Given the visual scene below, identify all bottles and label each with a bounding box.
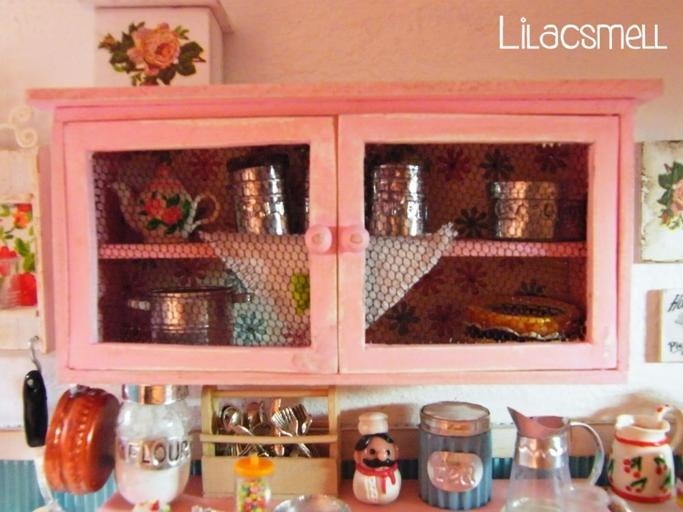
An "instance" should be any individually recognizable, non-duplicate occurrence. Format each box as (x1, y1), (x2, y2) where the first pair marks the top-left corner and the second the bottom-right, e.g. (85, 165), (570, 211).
(415, 396), (494, 510)
(505, 405), (606, 512)
(233, 451), (274, 512)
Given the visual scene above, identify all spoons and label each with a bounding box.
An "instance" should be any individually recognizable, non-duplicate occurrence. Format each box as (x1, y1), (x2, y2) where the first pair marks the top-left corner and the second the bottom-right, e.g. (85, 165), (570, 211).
(220, 400), (277, 456)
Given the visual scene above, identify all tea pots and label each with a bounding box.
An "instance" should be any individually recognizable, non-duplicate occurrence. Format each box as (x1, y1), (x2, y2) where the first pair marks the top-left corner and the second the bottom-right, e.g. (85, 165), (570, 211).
(605, 403), (683, 504)
(105, 161), (221, 243)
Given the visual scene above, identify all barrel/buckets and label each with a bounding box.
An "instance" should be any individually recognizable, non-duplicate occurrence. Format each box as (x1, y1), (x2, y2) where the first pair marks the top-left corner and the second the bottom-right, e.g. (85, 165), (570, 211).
(226, 153), (291, 235)
(366, 161), (429, 236)
(488, 180), (560, 242)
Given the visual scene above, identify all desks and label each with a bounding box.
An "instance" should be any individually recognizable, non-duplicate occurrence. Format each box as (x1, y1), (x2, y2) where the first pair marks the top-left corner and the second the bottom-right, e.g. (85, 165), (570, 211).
(96, 476), (682, 511)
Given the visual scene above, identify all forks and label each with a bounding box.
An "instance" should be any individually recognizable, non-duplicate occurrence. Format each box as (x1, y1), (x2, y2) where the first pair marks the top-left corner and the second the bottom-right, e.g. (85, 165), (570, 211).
(270, 403), (314, 458)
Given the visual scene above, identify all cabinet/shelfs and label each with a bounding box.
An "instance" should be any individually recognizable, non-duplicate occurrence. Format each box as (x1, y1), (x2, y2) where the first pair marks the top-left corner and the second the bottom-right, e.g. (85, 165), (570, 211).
(25, 78), (665, 389)
